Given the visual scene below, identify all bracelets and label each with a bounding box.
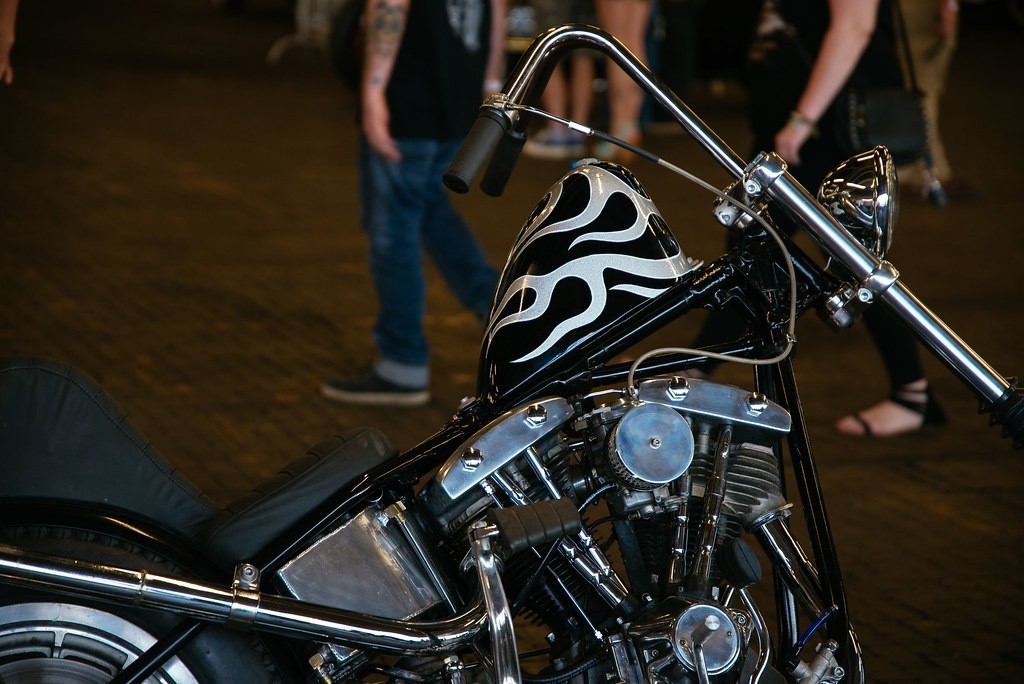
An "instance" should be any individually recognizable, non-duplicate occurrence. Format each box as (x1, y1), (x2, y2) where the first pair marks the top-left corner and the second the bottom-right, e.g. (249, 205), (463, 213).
(784, 108), (822, 138)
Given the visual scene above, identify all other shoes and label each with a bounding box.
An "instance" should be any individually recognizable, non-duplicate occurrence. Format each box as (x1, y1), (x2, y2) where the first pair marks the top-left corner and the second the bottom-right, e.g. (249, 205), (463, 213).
(591, 125), (641, 161)
(520, 126), (587, 160)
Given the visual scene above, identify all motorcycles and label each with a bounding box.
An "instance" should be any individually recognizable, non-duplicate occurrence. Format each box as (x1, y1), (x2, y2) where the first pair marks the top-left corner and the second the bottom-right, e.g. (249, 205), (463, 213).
(0, 22), (1024, 684)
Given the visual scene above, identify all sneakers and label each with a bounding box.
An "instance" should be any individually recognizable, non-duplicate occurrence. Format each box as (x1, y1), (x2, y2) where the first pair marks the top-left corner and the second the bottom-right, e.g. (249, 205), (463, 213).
(320, 366), (432, 407)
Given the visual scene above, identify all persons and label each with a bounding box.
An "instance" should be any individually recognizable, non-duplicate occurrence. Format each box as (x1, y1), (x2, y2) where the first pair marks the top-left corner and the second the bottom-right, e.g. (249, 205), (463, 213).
(263, 0), (972, 438)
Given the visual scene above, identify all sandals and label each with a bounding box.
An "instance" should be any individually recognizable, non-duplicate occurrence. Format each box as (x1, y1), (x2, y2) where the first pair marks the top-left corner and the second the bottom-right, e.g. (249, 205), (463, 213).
(833, 388), (948, 440)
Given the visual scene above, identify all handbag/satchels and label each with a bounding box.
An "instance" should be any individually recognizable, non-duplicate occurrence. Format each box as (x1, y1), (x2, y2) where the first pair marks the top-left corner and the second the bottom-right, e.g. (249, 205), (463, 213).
(833, 91), (928, 166)
